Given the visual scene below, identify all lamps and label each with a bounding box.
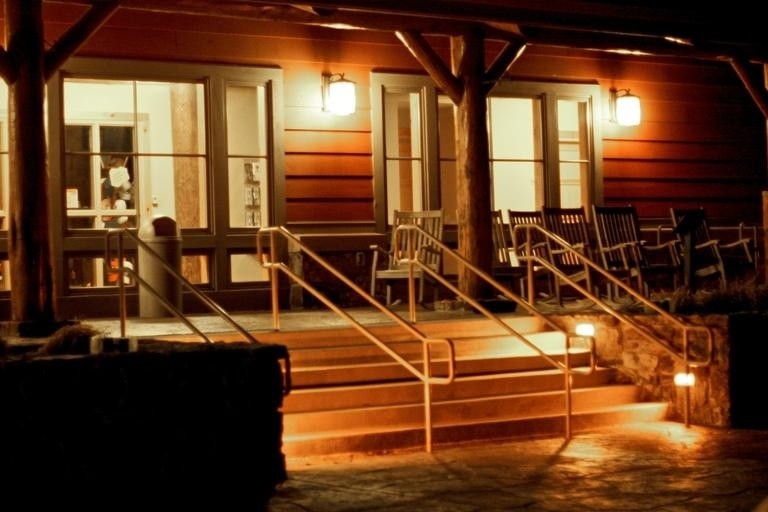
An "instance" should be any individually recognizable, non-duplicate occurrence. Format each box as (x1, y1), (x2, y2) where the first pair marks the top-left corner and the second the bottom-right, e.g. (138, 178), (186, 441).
(321, 71), (358, 116)
(608, 86), (642, 127)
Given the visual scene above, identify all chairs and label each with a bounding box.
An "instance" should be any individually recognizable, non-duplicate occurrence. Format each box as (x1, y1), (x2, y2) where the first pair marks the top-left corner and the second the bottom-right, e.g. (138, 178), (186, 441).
(369, 208), (445, 305)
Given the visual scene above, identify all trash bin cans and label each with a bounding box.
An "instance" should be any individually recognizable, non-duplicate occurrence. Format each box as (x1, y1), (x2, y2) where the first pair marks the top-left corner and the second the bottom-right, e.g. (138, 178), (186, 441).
(137, 215), (183, 320)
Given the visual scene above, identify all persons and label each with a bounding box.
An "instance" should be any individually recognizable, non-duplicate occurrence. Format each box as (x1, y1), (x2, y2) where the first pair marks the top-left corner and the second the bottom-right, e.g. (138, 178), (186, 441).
(33, 322), (120, 355)
